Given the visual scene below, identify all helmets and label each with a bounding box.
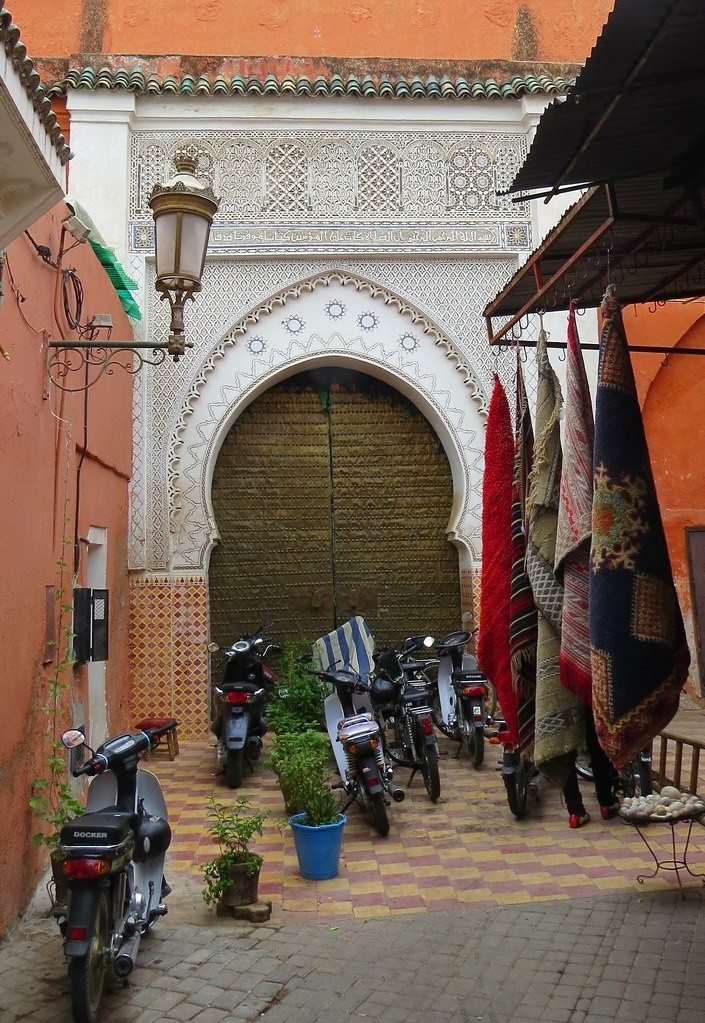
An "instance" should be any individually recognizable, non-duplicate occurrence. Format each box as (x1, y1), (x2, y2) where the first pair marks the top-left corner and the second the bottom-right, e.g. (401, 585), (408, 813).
(132, 814), (171, 863)
(370, 675), (397, 704)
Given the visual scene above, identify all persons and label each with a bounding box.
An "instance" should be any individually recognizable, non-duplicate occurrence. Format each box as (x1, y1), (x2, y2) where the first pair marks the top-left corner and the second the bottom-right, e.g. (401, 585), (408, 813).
(553, 728), (619, 827)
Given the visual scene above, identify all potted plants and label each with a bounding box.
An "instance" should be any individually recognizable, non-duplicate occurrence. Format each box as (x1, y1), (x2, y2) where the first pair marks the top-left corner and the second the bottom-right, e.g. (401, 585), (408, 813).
(198, 787), (271, 907)
(27, 491), (88, 905)
(262, 636), (330, 817)
(261, 728), (349, 881)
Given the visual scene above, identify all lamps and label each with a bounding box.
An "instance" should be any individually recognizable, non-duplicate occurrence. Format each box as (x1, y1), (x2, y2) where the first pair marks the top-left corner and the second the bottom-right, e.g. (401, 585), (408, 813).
(41, 147), (222, 401)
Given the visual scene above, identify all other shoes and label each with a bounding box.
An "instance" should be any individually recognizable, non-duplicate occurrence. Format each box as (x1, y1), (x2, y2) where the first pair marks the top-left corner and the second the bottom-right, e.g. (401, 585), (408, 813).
(600, 803), (620, 820)
(569, 812), (590, 828)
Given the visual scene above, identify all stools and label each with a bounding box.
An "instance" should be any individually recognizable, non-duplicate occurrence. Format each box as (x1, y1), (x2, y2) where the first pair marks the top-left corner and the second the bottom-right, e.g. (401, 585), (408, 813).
(135, 718), (179, 762)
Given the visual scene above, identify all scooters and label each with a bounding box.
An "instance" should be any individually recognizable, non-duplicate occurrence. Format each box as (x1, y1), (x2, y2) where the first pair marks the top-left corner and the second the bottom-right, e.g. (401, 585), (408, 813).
(368, 611), (490, 803)
(207, 634), (280, 790)
(489, 708), (654, 825)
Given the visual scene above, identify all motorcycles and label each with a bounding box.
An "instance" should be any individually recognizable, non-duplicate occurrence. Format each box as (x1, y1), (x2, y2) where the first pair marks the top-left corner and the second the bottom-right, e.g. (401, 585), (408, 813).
(307, 659), (405, 838)
(46, 717), (180, 1023)
